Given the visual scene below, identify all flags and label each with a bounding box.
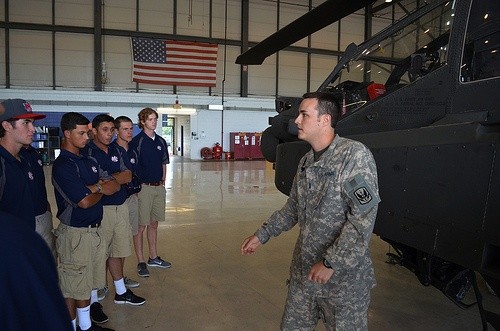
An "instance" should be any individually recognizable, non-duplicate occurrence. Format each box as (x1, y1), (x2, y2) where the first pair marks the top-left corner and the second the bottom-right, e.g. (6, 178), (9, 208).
(131, 35), (217, 87)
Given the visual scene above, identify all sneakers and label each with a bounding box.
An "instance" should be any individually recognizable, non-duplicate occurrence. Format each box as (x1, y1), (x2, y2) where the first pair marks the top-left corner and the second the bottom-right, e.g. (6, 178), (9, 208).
(147, 256), (172, 268)
(137, 262), (150, 276)
(114, 288), (145, 306)
(124, 277), (140, 287)
(97, 287), (109, 300)
(90, 302), (109, 324)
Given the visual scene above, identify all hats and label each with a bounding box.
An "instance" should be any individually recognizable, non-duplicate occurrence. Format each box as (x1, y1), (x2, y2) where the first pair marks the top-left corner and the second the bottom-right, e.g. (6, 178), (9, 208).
(0, 98), (46, 122)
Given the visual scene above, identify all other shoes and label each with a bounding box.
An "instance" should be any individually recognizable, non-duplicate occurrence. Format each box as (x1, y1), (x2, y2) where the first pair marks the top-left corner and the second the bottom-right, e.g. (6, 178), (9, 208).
(76, 321), (115, 331)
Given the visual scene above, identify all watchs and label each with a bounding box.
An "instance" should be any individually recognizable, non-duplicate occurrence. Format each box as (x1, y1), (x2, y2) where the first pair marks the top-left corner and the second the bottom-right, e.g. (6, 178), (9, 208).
(322, 258), (333, 269)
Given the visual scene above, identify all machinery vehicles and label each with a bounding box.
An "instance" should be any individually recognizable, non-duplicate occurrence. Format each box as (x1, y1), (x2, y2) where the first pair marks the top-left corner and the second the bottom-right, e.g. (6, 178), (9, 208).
(235, 0), (500, 331)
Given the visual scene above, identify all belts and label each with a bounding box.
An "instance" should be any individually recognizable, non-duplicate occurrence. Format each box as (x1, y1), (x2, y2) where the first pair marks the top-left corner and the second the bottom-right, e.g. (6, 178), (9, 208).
(144, 181), (163, 186)
(89, 220), (102, 230)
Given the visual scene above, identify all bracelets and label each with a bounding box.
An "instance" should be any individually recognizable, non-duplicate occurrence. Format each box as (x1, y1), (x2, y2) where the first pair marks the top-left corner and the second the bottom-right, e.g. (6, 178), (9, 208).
(96, 184), (102, 193)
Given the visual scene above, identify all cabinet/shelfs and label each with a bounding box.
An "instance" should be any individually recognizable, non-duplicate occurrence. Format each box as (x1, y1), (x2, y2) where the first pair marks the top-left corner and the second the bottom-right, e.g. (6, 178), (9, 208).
(230, 131), (265, 162)
(33, 126), (60, 166)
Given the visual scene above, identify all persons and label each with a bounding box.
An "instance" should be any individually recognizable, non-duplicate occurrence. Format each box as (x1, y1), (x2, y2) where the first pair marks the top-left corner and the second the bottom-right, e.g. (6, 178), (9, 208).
(97, 115), (139, 301)
(0, 211), (74, 331)
(50, 112), (120, 331)
(129, 107), (172, 277)
(0, 98), (47, 232)
(89, 114), (146, 324)
(240, 92), (382, 331)
(18, 143), (58, 263)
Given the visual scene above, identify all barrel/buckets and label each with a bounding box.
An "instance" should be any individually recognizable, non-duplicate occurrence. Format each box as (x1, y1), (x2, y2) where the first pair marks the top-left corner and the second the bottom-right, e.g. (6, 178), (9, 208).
(226, 153), (231, 160)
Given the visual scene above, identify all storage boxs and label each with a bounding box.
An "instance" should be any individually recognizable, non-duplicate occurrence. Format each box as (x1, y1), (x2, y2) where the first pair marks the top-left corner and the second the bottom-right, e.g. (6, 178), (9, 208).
(366, 83), (386, 101)
